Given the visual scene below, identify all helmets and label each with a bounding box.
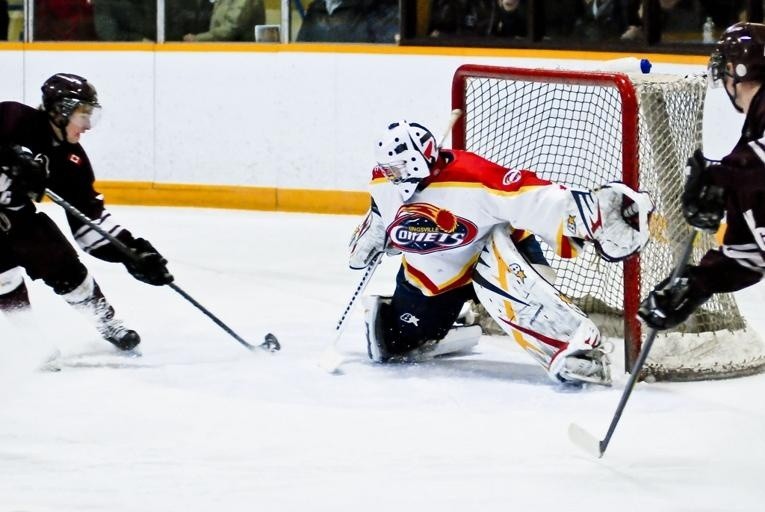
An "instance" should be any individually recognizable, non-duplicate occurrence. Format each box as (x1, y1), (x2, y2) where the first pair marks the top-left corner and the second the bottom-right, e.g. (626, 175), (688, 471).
(706, 20), (764, 112)
(42, 70), (102, 133)
(375, 119), (437, 202)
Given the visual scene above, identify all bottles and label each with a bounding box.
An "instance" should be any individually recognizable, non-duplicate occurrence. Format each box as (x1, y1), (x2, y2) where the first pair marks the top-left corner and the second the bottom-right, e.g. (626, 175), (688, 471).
(702, 17), (716, 44)
(593, 56), (654, 76)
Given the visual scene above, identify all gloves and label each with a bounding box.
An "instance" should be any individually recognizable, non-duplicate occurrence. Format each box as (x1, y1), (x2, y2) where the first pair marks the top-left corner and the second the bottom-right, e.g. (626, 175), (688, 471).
(681, 147), (729, 235)
(635, 262), (715, 331)
(11, 146), (46, 202)
(124, 235), (174, 284)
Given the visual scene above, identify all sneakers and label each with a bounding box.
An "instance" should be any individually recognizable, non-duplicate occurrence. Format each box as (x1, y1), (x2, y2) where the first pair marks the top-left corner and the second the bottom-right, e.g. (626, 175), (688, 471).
(99, 319), (140, 352)
(557, 352), (602, 388)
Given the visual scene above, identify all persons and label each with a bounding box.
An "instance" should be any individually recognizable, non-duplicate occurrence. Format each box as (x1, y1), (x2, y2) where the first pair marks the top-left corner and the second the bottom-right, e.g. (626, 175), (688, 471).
(295, 2), (398, 42)
(632, 21), (765, 331)
(427, 3), (762, 48)
(0, 4), (266, 41)
(0, 72), (175, 352)
(337, 117), (659, 392)
